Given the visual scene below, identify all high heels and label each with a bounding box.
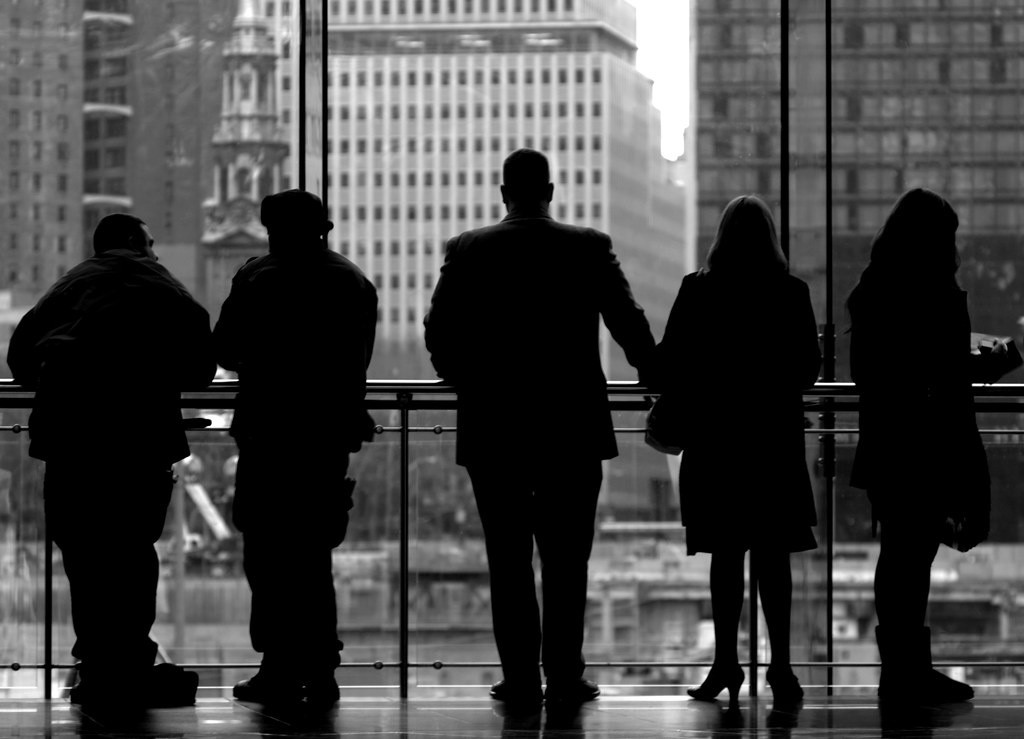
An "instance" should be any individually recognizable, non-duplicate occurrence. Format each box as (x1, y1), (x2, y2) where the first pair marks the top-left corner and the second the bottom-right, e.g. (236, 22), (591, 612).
(765, 667), (803, 705)
(689, 662), (744, 704)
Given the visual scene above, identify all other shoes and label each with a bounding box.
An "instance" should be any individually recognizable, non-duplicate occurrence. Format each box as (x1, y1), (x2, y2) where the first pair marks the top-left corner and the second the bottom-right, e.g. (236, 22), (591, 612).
(545, 676), (597, 700)
(493, 679), (544, 703)
(923, 668), (974, 705)
(234, 681), (299, 707)
(877, 688), (954, 727)
(304, 673), (339, 702)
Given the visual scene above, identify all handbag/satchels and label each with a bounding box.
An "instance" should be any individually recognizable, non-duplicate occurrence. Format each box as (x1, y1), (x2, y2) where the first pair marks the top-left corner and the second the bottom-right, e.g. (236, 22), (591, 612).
(645, 383), (697, 455)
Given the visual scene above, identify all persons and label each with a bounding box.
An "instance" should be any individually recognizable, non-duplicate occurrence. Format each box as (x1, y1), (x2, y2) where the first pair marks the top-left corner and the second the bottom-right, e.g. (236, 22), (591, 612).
(7, 213), (217, 702)
(659, 197), (822, 704)
(423, 150), (656, 703)
(213, 189), (378, 700)
(845, 189), (1008, 704)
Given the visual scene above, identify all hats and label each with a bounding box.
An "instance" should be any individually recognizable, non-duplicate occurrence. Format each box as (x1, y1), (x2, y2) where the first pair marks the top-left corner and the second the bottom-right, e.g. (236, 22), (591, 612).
(259, 189), (333, 234)
